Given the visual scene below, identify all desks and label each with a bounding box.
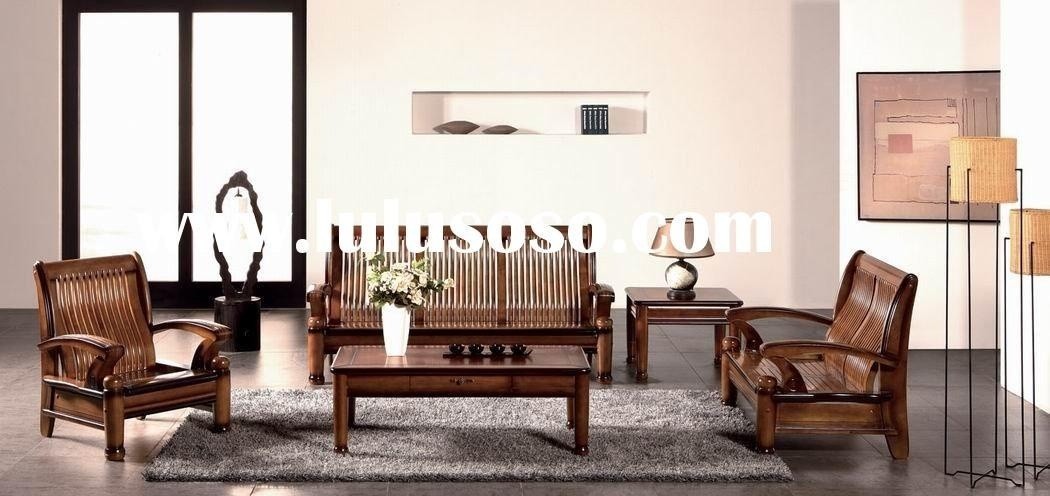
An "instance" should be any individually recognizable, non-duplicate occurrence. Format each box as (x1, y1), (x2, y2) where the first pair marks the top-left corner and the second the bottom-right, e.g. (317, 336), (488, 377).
(623, 284), (746, 383)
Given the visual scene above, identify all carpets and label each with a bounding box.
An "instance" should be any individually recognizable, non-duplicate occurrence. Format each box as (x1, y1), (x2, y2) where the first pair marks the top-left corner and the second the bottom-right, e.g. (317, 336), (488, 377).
(142, 382), (798, 486)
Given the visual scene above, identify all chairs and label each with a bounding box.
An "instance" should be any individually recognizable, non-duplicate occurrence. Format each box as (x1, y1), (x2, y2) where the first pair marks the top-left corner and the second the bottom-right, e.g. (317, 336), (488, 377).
(30, 249), (236, 463)
(717, 248), (920, 463)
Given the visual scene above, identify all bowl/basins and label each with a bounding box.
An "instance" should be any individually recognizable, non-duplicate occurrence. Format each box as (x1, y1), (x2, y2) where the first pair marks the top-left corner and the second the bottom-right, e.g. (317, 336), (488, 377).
(468, 344), (484, 353)
(510, 343), (527, 353)
(449, 344), (465, 353)
(489, 343), (506, 353)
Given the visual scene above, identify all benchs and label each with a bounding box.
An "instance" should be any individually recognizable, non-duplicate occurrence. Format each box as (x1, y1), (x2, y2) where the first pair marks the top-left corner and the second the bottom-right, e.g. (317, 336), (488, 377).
(303, 213), (617, 391)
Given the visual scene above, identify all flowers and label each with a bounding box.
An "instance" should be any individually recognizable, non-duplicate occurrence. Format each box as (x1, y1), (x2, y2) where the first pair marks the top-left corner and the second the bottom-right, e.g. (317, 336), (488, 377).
(358, 248), (458, 309)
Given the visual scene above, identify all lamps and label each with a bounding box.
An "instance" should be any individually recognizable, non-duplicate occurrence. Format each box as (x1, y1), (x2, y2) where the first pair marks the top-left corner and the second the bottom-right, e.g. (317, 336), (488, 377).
(1000, 202), (1050, 478)
(937, 129), (1025, 489)
(646, 212), (718, 292)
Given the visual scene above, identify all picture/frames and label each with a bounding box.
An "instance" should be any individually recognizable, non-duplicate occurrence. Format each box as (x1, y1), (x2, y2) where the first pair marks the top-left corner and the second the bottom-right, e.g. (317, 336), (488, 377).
(853, 66), (1000, 228)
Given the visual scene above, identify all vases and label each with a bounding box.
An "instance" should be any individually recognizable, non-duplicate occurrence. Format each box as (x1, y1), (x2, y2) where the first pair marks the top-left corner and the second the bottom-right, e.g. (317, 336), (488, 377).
(377, 294), (413, 357)
